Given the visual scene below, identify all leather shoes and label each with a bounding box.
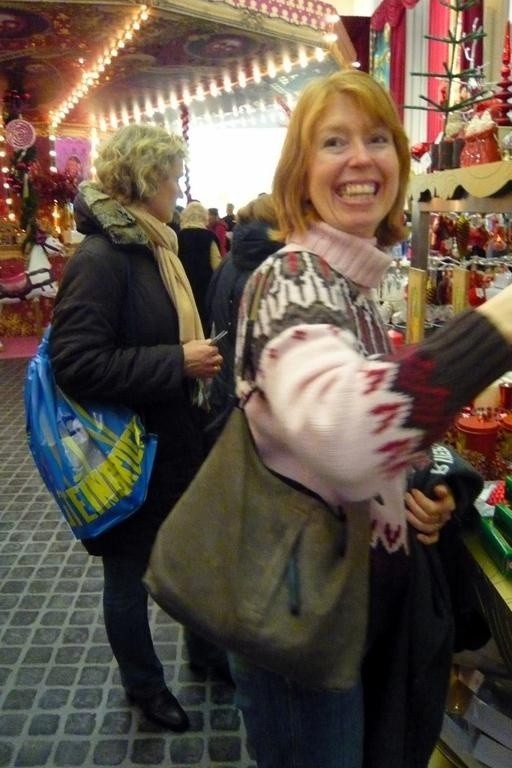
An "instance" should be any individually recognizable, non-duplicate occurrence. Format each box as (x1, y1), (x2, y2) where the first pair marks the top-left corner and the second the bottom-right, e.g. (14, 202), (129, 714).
(125, 685), (189, 733)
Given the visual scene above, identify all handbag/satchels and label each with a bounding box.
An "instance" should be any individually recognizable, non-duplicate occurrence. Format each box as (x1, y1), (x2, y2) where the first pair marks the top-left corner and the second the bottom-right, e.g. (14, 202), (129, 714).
(143, 400), (371, 696)
(22, 322), (161, 541)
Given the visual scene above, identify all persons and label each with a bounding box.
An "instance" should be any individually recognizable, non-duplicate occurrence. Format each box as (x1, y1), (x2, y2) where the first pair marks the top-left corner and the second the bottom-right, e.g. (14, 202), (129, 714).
(48, 123), (238, 733)
(230, 72), (512, 768)
(171, 192), (284, 404)
